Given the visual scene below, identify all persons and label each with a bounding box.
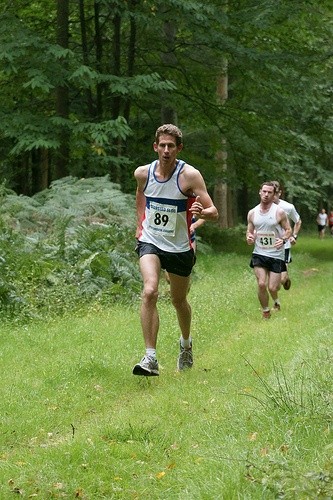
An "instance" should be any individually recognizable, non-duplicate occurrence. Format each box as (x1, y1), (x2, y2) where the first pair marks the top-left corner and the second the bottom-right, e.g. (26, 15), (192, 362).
(133, 124), (219, 375)
(247, 180), (302, 319)
(317, 208), (328, 238)
(328, 211), (333, 234)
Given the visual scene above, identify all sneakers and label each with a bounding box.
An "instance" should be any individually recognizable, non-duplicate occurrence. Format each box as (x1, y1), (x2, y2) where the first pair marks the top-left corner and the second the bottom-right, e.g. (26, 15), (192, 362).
(270, 302), (280, 311)
(262, 310), (270, 319)
(178, 337), (193, 370)
(283, 278), (291, 290)
(132, 355), (159, 376)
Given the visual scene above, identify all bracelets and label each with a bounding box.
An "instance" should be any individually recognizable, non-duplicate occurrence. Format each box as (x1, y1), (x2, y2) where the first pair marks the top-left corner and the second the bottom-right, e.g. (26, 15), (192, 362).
(291, 235), (298, 240)
(281, 237), (286, 245)
